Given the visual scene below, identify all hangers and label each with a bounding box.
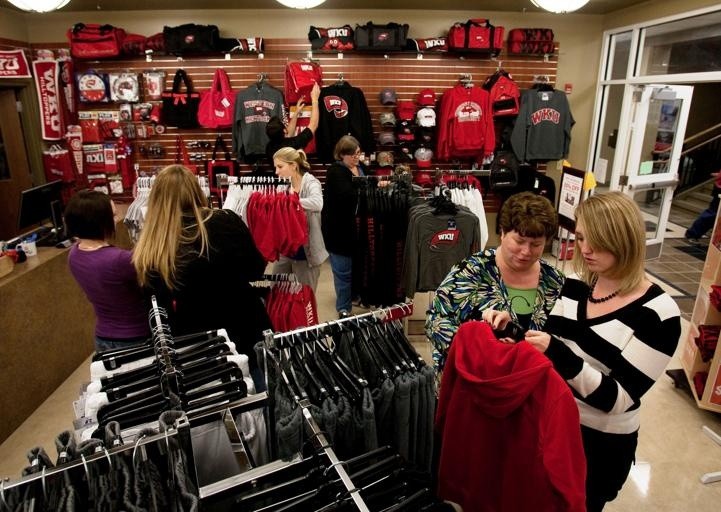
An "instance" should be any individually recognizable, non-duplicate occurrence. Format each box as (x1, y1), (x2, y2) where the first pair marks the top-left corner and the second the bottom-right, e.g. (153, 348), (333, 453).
(136, 174), (292, 209)
(460, 73), (475, 90)
(529, 74), (556, 95)
(0, 295), (443, 512)
(352, 166), (492, 217)
(490, 294), (531, 342)
(255, 73), (265, 87)
(253, 267), (305, 295)
(334, 72), (346, 87)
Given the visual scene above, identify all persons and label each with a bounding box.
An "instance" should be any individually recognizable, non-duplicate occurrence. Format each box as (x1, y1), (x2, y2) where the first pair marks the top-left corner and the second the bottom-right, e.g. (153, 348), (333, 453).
(65, 190), (152, 347)
(321, 137), (372, 318)
(422, 195), (562, 388)
(268, 149), (327, 297)
(133, 164), (277, 387)
(526, 190), (681, 512)
(682, 168), (721, 245)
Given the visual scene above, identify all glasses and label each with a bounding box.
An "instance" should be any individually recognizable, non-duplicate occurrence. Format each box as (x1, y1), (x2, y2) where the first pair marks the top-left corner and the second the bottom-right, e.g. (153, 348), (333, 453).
(343, 151), (360, 157)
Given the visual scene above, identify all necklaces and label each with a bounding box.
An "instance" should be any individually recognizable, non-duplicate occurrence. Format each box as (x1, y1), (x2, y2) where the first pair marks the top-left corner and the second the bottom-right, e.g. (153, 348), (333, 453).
(589, 273), (623, 304)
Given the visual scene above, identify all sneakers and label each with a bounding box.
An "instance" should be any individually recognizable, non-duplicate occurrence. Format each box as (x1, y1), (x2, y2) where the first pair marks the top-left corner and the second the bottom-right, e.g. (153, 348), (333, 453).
(685, 236), (700, 245)
(700, 234), (709, 238)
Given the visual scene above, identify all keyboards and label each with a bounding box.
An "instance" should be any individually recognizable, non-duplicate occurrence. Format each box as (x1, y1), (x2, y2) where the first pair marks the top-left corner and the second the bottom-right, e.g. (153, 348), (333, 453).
(6, 227), (55, 250)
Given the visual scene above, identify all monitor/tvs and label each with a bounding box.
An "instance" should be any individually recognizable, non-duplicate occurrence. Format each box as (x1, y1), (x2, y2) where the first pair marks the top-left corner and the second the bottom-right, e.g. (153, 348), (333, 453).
(18, 179), (73, 248)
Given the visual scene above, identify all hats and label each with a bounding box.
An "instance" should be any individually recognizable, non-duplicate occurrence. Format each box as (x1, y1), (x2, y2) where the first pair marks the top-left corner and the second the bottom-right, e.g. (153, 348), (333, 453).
(379, 133), (395, 145)
(377, 153), (394, 167)
(394, 122), (415, 142)
(417, 90), (437, 105)
(417, 134), (432, 147)
(374, 170), (395, 187)
(418, 107), (436, 127)
(416, 172), (432, 184)
(381, 89), (397, 105)
(394, 100), (417, 121)
(414, 149), (433, 168)
(379, 113), (396, 128)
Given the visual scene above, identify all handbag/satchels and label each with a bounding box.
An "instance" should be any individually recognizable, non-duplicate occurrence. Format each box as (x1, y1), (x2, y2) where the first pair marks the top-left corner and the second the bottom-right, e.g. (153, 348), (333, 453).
(125, 32), (165, 55)
(163, 24), (220, 55)
(308, 25), (354, 50)
(67, 22), (125, 60)
(197, 69), (239, 128)
(227, 38), (264, 53)
(508, 28), (555, 56)
(354, 22), (409, 50)
(449, 18), (504, 50)
(208, 138), (238, 196)
(162, 70), (200, 129)
(409, 37), (450, 50)
